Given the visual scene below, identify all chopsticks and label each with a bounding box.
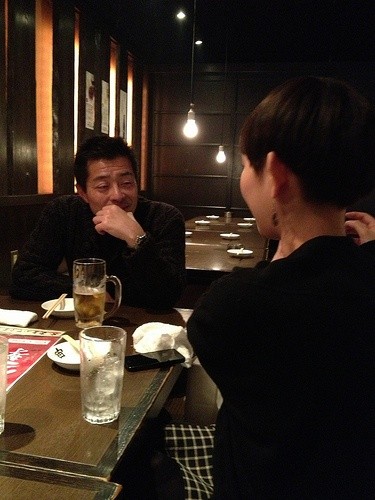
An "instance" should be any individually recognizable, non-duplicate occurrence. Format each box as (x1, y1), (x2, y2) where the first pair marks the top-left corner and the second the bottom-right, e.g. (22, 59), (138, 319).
(237, 247), (244, 255)
(60, 335), (80, 352)
(42, 294), (67, 319)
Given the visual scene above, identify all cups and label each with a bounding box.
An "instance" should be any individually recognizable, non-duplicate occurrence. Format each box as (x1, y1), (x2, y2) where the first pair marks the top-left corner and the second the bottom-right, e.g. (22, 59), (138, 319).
(78, 325), (127, 425)
(0, 334), (9, 434)
(73, 257), (122, 330)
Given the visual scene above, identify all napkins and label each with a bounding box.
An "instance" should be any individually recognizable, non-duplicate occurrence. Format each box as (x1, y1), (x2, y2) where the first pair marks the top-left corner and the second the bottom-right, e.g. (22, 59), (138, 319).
(0, 309), (38, 328)
(131, 322), (194, 368)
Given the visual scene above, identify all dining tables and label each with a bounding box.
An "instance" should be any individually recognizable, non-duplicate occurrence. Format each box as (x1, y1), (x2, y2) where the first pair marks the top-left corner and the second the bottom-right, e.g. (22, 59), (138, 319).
(0, 291), (188, 482)
(184, 215), (270, 307)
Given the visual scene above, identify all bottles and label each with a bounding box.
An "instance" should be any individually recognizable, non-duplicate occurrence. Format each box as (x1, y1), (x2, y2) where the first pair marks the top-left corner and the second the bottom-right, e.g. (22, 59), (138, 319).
(224, 207), (231, 225)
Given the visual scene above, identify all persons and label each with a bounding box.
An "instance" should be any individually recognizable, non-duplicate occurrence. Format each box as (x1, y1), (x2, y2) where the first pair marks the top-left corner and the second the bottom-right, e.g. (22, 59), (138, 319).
(146, 77), (375, 500)
(9, 137), (186, 312)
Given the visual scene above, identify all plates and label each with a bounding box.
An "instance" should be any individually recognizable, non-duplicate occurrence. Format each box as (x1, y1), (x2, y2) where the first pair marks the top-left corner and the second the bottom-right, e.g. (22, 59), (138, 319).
(206, 216), (220, 220)
(237, 223), (254, 229)
(220, 233), (240, 239)
(185, 231), (192, 237)
(195, 220), (211, 226)
(226, 248), (254, 257)
(244, 218), (256, 221)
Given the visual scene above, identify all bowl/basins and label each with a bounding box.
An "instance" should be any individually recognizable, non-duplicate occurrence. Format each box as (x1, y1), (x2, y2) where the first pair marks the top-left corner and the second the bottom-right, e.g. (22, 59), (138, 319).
(42, 298), (75, 318)
(47, 337), (111, 371)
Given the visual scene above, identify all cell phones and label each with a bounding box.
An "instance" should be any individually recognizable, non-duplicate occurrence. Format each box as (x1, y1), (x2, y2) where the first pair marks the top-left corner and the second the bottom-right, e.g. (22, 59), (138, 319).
(125, 348), (185, 372)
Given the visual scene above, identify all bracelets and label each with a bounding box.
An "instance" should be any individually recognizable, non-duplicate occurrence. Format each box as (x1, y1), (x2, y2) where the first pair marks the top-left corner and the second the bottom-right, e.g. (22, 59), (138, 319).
(135, 234), (147, 249)
